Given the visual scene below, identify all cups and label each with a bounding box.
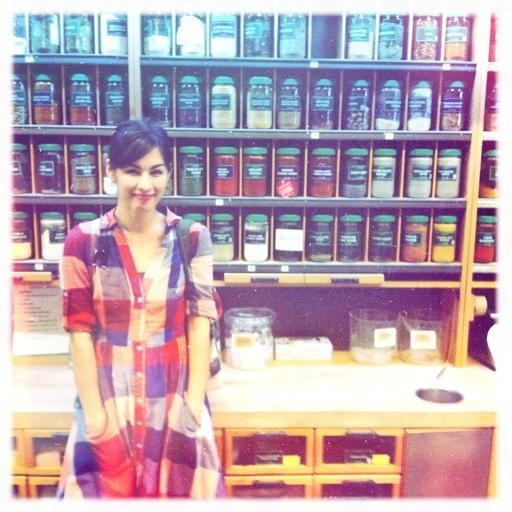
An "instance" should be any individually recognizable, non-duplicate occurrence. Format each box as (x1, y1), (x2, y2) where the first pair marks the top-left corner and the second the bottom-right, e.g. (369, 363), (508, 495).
(399, 307), (450, 364)
(348, 306), (400, 366)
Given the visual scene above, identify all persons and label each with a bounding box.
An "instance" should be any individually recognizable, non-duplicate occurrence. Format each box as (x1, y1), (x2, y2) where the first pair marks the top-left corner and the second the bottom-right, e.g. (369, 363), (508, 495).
(53, 118), (227, 499)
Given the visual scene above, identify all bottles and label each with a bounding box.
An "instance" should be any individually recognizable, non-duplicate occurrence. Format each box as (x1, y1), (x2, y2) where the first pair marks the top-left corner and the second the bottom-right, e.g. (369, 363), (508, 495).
(375, 80), (401, 131)
(445, 18), (470, 61)
(432, 214), (457, 265)
(14, 145), (29, 194)
(245, 76), (273, 128)
(412, 16), (439, 61)
(279, 79), (301, 128)
(35, 144), (63, 196)
(407, 150), (433, 197)
(141, 14), (173, 58)
(64, 14), (94, 56)
(273, 147), (299, 198)
(176, 77), (203, 129)
(14, 66), (27, 125)
(99, 16), (127, 58)
(477, 215), (494, 263)
(309, 216), (333, 260)
(76, 212), (96, 221)
(214, 148), (238, 197)
(242, 214), (268, 263)
(371, 216), (394, 262)
(240, 146), (267, 196)
(209, 13), (237, 60)
(176, 15), (205, 58)
(401, 214), (428, 261)
(489, 14), (496, 60)
(346, 15), (375, 61)
(378, 16), (403, 61)
(336, 215), (363, 260)
(185, 212), (206, 222)
(443, 81), (462, 132)
(69, 144), (95, 196)
(146, 78), (171, 126)
(209, 76), (239, 130)
(273, 214), (302, 260)
(103, 75), (127, 124)
(101, 145), (113, 195)
(213, 215), (233, 264)
(244, 18), (271, 58)
(310, 78), (335, 129)
(14, 16), (29, 59)
(33, 71), (60, 122)
(480, 150), (496, 199)
(371, 149), (396, 199)
(31, 14), (62, 56)
(280, 16), (306, 59)
(310, 148), (335, 196)
(342, 149), (368, 197)
(220, 307), (275, 371)
(67, 73), (95, 125)
(12, 213), (32, 260)
(485, 74), (498, 129)
(345, 79), (368, 133)
(39, 213), (68, 260)
(409, 81), (432, 136)
(437, 150), (461, 199)
(179, 147), (203, 198)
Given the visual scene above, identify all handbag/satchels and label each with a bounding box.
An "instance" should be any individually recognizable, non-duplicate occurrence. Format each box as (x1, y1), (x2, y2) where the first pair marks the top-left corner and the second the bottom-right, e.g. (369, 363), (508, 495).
(175, 219), (222, 378)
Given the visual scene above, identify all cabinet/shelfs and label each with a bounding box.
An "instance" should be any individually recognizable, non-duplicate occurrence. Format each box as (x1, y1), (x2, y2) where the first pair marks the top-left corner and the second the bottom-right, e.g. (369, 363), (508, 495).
(12, 410), (497, 498)
(12, 15), (494, 366)
